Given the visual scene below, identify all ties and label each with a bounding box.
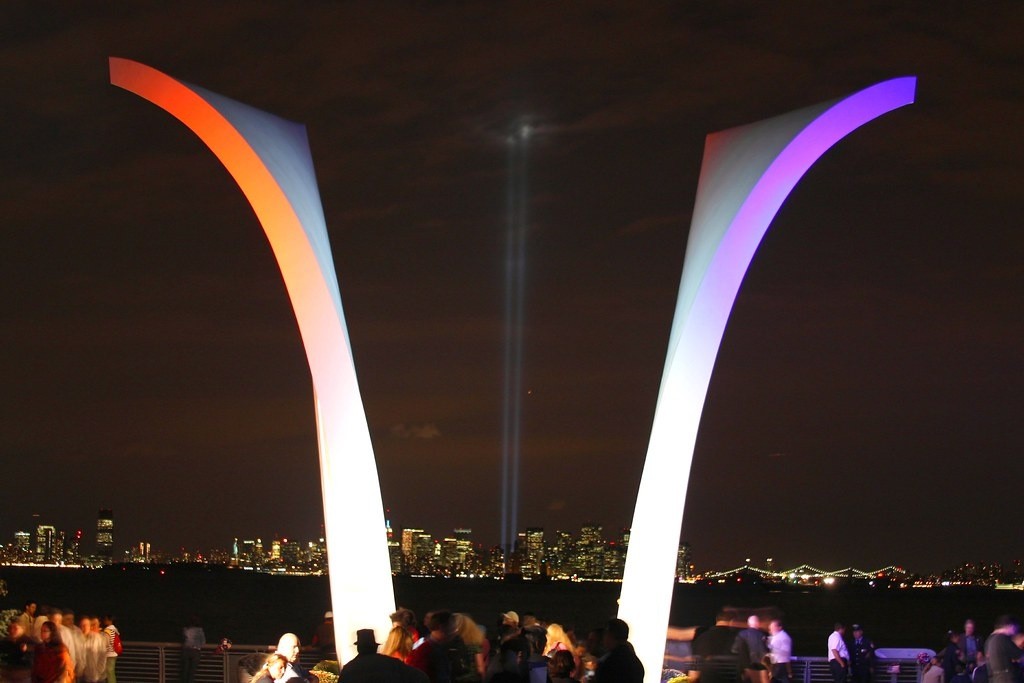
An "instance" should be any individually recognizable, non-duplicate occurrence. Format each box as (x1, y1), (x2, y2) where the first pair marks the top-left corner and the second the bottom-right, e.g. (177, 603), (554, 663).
(857, 640), (859, 646)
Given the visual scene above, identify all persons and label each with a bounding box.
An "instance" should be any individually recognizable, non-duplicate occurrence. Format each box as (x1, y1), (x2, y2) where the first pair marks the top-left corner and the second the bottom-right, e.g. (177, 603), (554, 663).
(922, 619), (1024, 683)
(0, 602), (120, 683)
(249, 609), (643, 683)
(827, 623), (874, 683)
(732, 615), (792, 683)
(179, 615), (205, 683)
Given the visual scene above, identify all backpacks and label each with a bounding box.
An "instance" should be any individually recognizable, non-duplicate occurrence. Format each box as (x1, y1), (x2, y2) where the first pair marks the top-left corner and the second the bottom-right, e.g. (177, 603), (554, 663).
(114, 631), (124, 655)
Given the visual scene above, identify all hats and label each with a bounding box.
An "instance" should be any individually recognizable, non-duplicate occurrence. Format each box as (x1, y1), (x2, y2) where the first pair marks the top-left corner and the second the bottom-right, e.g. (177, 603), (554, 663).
(325, 611), (333, 619)
(424, 610), (450, 625)
(503, 611), (519, 623)
(355, 629), (380, 646)
(849, 624), (862, 631)
